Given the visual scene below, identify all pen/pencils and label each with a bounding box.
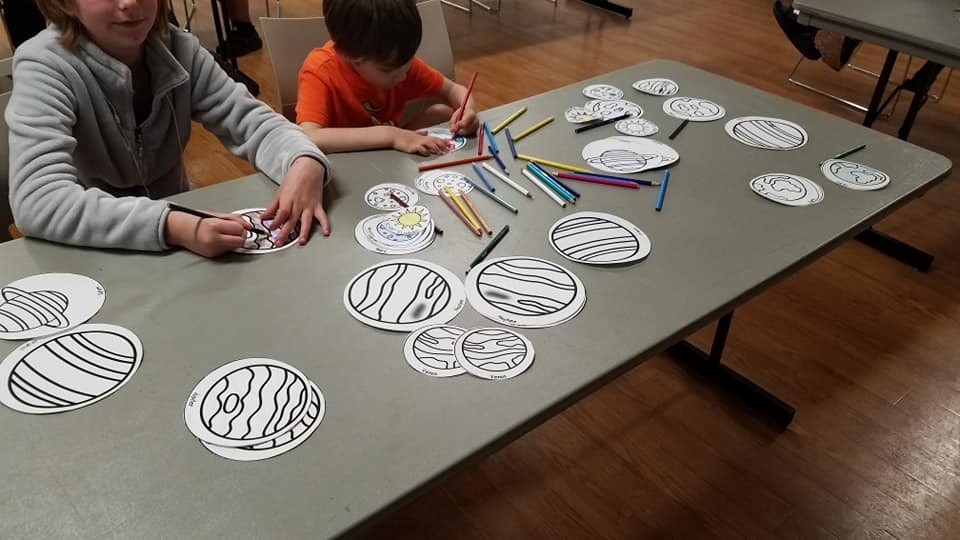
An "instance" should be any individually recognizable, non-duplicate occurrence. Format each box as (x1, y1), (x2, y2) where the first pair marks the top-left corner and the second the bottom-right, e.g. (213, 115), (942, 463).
(551, 170), (641, 190)
(487, 144), (510, 175)
(491, 106), (527, 134)
(669, 118), (689, 139)
(526, 161), (581, 205)
(574, 171), (661, 186)
(439, 185), (482, 236)
(482, 120), (499, 152)
(166, 204), (270, 235)
(449, 70), (478, 140)
(458, 189), (492, 234)
(515, 153), (593, 174)
(505, 125), (518, 159)
(464, 176), (519, 213)
(656, 169), (670, 211)
(472, 161), (495, 192)
(482, 163), (535, 200)
(418, 153), (494, 171)
(464, 225), (509, 272)
(521, 167), (567, 208)
(819, 144), (867, 165)
(512, 116), (555, 141)
(575, 113), (631, 133)
(477, 120), (484, 154)
(390, 194), (443, 234)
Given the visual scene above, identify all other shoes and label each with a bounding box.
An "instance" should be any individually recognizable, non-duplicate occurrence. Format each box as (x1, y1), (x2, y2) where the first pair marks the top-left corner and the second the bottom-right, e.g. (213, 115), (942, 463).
(216, 19), (263, 59)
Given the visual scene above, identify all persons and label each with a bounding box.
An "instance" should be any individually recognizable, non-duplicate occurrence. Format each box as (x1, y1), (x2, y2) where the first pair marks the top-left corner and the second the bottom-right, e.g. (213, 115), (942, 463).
(4, 0), (331, 258)
(295, 0), (479, 156)
(220, 0), (263, 58)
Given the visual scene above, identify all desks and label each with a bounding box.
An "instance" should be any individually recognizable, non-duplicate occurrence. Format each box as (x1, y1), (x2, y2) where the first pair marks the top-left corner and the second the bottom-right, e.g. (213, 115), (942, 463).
(794, 0), (960, 272)
(0, 59), (951, 540)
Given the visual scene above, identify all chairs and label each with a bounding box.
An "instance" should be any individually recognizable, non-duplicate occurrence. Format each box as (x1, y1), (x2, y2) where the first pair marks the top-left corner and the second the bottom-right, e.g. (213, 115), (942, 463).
(788, 31), (953, 118)
(260, 0), (456, 121)
